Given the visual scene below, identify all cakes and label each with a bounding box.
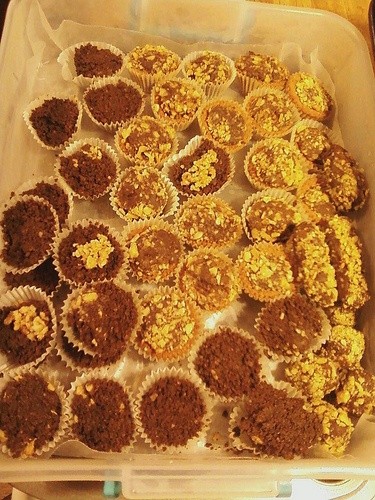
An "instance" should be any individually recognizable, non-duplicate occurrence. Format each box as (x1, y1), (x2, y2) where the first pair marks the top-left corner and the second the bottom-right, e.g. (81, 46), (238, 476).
(0, 45), (375, 459)
